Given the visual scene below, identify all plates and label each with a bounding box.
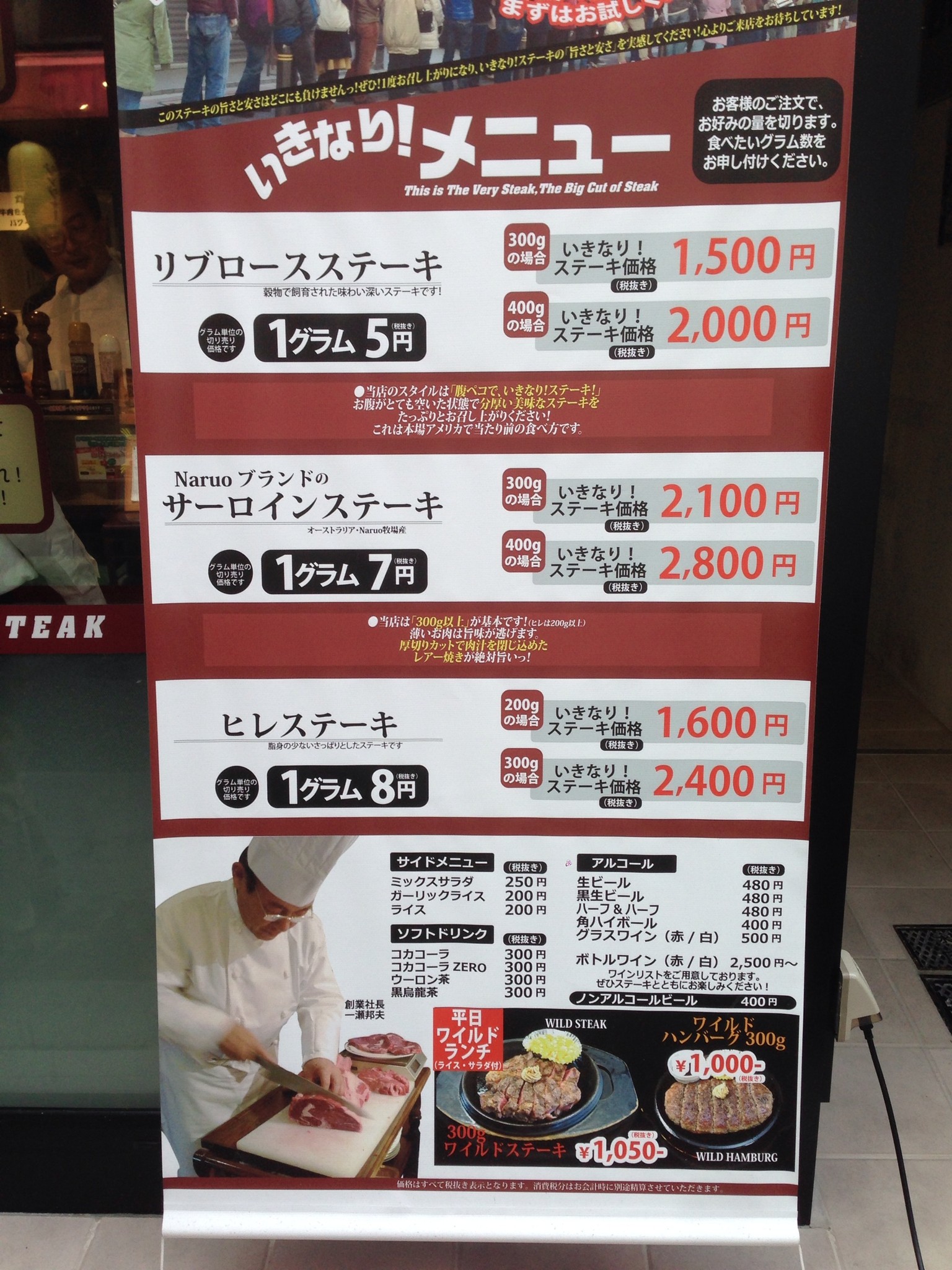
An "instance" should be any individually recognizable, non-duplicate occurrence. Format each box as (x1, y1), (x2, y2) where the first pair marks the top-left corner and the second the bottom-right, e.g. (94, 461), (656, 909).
(345, 1041), (413, 1059)
(458, 1040), (605, 1137)
(382, 1127), (403, 1163)
(657, 1065), (781, 1148)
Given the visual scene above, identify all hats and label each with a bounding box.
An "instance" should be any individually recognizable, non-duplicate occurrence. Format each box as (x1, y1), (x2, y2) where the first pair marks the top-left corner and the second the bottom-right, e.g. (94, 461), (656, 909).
(247, 835), (360, 907)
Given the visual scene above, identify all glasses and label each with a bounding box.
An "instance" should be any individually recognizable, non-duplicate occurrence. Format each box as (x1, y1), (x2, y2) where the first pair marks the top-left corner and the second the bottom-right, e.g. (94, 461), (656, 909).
(252, 886), (316, 923)
(33, 210), (97, 249)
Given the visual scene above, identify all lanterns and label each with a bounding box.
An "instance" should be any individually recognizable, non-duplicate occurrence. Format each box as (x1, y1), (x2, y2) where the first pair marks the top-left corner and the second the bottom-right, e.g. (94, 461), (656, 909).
(10, 138), (61, 239)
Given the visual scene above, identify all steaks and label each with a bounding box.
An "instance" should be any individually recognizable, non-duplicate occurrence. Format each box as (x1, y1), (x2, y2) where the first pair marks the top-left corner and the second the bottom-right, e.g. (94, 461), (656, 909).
(663, 1078), (773, 1136)
(478, 1051), (582, 1122)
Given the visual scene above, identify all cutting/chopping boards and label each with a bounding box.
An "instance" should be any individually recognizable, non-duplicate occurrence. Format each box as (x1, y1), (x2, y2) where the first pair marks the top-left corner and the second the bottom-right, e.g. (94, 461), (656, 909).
(237, 1074), (414, 1178)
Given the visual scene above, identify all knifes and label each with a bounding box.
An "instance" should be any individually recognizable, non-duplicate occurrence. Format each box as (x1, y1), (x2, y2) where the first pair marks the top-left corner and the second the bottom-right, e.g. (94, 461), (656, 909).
(252, 1053), (375, 1121)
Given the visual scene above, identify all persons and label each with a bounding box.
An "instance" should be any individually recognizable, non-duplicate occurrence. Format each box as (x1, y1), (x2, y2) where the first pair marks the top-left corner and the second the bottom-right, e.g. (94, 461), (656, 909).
(154, 837), (361, 1177)
(15, 170), (129, 389)
(0, 500), (107, 605)
(114, 0), (857, 138)
